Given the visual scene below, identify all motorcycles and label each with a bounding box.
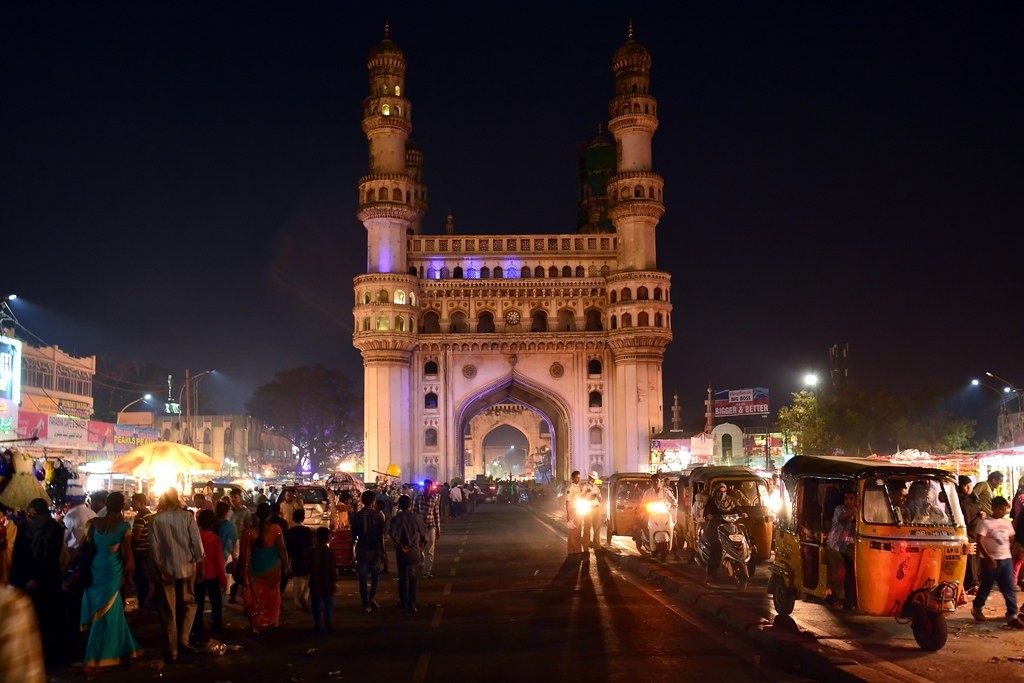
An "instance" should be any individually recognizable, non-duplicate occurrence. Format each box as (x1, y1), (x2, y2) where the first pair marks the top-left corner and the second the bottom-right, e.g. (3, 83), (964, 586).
(689, 465), (773, 577)
(603, 473), (654, 543)
(580, 481), (609, 519)
(673, 477), (690, 548)
(767, 455), (978, 651)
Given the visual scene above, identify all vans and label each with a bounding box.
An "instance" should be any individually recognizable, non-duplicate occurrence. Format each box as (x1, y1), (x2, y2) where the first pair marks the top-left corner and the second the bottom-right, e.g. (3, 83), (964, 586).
(275, 486), (337, 532)
(191, 483), (258, 514)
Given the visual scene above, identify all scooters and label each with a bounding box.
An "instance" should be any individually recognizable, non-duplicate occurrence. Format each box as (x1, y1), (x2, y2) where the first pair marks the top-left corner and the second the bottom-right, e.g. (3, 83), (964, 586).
(631, 500), (679, 563)
(695, 510), (752, 592)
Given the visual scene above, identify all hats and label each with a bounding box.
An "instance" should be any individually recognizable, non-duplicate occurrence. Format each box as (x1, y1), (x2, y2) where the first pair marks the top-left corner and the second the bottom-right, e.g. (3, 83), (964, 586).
(65, 479), (88, 501)
(566, 519), (577, 530)
(588, 470), (598, 480)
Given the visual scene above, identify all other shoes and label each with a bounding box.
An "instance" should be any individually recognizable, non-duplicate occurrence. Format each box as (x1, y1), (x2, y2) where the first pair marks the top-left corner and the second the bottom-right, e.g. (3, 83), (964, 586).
(970, 606), (987, 622)
(313, 622), (324, 634)
(569, 549), (605, 558)
(1005, 615), (1024, 628)
(421, 573), (434, 580)
(362, 602), (371, 611)
(401, 603), (410, 613)
(409, 604), (419, 612)
(180, 638), (196, 649)
(323, 615), (334, 631)
(369, 598), (380, 609)
(169, 654), (179, 662)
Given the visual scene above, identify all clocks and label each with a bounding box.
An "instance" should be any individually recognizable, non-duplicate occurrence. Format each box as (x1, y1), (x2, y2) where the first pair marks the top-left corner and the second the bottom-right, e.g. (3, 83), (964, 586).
(506, 311), (520, 325)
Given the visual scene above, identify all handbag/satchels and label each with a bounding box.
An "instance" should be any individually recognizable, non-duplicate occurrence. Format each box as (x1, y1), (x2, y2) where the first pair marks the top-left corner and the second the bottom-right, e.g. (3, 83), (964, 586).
(226, 560), (237, 574)
(62, 540), (97, 589)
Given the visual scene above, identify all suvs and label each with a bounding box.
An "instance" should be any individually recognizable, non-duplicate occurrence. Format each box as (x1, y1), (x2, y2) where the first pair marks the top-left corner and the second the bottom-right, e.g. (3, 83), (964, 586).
(476, 482), (498, 503)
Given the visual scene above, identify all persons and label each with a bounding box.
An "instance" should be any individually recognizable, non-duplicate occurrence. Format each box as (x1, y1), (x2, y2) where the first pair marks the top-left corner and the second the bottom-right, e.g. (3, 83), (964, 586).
(0, 480), (497, 667)
(619, 474), (678, 525)
(827, 491), (856, 611)
(971, 496), (1024, 628)
(497, 483), (504, 505)
(582, 471), (602, 555)
(0, 526), (47, 683)
(955, 475), (979, 595)
(1010, 476), (1024, 592)
(902, 480), (949, 524)
(511, 481), (518, 504)
(692, 483), (748, 585)
(759, 478), (780, 511)
(566, 471), (583, 556)
(973, 471), (1004, 516)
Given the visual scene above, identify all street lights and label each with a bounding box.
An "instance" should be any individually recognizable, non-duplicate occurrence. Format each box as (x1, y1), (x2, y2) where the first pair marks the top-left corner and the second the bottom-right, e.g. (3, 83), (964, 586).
(178, 369), (216, 444)
(117, 394), (151, 425)
(986, 371), (1022, 434)
(804, 374), (819, 434)
(971, 379), (1013, 441)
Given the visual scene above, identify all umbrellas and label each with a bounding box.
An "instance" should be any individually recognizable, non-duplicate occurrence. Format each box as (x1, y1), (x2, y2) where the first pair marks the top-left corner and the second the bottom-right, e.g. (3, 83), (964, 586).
(112, 441), (221, 492)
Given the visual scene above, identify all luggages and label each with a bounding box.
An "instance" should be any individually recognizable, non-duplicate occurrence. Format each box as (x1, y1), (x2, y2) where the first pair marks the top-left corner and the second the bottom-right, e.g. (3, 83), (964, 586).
(330, 530), (358, 577)
